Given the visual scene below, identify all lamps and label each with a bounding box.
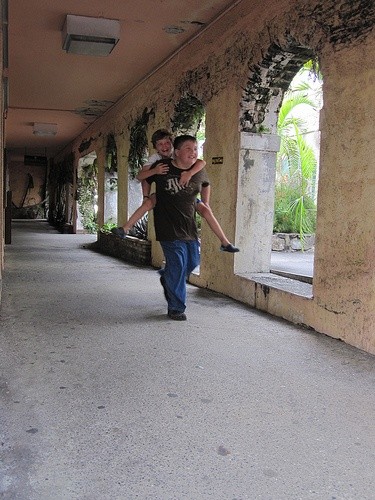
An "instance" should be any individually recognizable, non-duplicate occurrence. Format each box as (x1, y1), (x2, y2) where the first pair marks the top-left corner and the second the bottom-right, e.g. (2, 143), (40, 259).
(59, 15), (122, 58)
(31, 122), (57, 136)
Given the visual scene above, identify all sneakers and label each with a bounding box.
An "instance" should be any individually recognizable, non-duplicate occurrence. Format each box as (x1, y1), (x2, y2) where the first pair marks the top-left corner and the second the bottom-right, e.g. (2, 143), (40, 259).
(168, 309), (186, 321)
(221, 243), (240, 253)
(160, 277), (168, 301)
(111, 227), (126, 239)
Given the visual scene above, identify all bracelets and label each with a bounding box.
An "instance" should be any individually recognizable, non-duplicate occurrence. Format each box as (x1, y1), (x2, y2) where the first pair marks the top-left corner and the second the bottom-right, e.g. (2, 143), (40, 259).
(143, 194), (149, 199)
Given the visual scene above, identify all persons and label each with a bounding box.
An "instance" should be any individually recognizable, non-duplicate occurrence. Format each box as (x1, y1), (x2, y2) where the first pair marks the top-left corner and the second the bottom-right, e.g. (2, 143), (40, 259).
(141, 135), (211, 321)
(109, 127), (241, 254)
